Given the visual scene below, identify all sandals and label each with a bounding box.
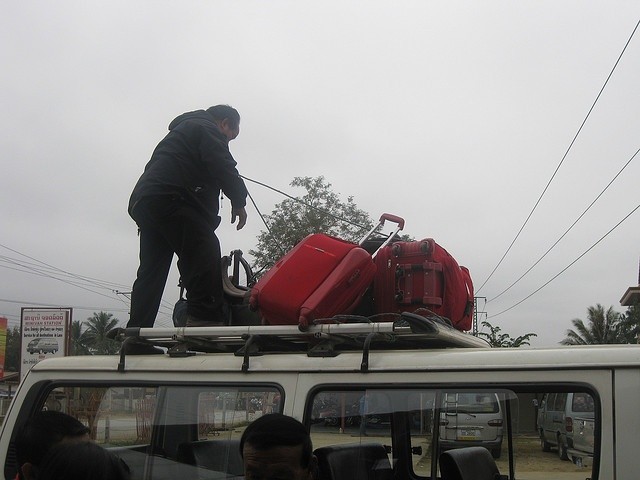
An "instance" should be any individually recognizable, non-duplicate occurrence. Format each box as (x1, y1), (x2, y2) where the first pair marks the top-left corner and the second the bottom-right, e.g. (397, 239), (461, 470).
(348, 234), (403, 322)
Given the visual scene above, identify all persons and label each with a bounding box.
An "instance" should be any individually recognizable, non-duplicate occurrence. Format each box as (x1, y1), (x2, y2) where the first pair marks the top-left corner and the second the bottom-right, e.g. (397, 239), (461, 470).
(36, 441), (131, 480)
(118, 105), (248, 356)
(14, 409), (92, 480)
(239, 413), (312, 480)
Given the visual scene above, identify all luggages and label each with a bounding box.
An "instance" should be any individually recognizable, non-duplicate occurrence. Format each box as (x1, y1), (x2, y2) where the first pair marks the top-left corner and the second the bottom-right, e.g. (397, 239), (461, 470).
(374, 239), (473, 332)
(248, 213), (404, 332)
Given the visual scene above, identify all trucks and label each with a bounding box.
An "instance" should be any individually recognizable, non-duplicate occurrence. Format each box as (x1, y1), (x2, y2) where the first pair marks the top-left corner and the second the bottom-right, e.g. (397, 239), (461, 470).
(2, 310), (640, 480)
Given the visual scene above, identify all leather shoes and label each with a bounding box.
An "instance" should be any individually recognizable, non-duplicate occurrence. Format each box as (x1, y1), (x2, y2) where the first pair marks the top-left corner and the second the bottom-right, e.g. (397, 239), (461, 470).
(119, 342), (164, 356)
(187, 312), (229, 327)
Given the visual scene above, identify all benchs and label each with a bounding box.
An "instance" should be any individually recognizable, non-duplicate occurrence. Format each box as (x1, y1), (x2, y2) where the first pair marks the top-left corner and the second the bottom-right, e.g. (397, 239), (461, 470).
(439, 447), (508, 479)
(314, 441), (393, 480)
(179, 439), (245, 477)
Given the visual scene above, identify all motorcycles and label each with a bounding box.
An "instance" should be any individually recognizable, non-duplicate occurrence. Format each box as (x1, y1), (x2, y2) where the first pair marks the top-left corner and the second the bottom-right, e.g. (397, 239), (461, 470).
(208, 389), (394, 427)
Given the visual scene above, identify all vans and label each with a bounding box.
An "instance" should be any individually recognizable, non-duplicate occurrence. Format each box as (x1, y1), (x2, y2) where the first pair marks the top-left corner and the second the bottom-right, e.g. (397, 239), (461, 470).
(25, 336), (60, 354)
(531, 388), (595, 462)
(429, 386), (506, 462)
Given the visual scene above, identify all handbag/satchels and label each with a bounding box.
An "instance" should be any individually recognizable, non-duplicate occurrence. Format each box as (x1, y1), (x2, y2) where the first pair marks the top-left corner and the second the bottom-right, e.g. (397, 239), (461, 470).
(173, 287), (231, 341)
